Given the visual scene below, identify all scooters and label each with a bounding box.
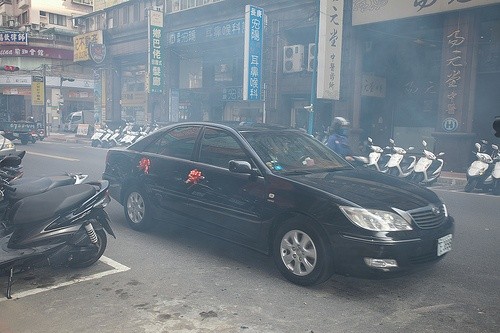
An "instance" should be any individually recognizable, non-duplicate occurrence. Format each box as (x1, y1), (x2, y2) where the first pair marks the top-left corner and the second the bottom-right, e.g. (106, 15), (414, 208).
(1, 130), (117, 300)
(352, 136), (500, 196)
(90, 121), (164, 148)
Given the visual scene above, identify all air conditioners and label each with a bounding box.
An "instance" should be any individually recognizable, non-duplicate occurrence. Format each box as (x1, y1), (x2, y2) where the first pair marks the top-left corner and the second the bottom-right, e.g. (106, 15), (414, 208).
(308, 43), (318, 73)
(31, 23), (40, 30)
(284, 44), (304, 73)
(41, 22), (48, 28)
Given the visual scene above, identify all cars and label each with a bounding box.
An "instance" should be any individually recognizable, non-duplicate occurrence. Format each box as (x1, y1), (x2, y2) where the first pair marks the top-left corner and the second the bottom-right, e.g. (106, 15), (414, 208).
(99, 121), (456, 289)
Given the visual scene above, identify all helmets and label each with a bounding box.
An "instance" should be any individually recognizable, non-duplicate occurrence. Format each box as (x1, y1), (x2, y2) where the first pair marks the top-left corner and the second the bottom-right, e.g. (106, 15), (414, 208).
(333, 117), (350, 136)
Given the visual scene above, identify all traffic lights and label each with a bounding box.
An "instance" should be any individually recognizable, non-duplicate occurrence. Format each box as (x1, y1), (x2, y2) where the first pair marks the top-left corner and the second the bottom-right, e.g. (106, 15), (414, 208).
(3, 65), (19, 72)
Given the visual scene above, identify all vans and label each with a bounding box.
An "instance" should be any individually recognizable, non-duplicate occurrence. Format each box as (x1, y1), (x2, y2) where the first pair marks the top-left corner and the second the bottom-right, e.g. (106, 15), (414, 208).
(62, 109), (98, 133)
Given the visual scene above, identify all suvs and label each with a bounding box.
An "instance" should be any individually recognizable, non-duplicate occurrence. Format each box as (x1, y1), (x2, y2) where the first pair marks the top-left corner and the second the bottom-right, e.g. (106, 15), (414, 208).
(0, 118), (45, 145)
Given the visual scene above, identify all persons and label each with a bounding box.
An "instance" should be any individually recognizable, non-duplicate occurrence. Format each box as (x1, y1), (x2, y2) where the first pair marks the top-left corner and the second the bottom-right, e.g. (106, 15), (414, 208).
(329, 118), (355, 162)
